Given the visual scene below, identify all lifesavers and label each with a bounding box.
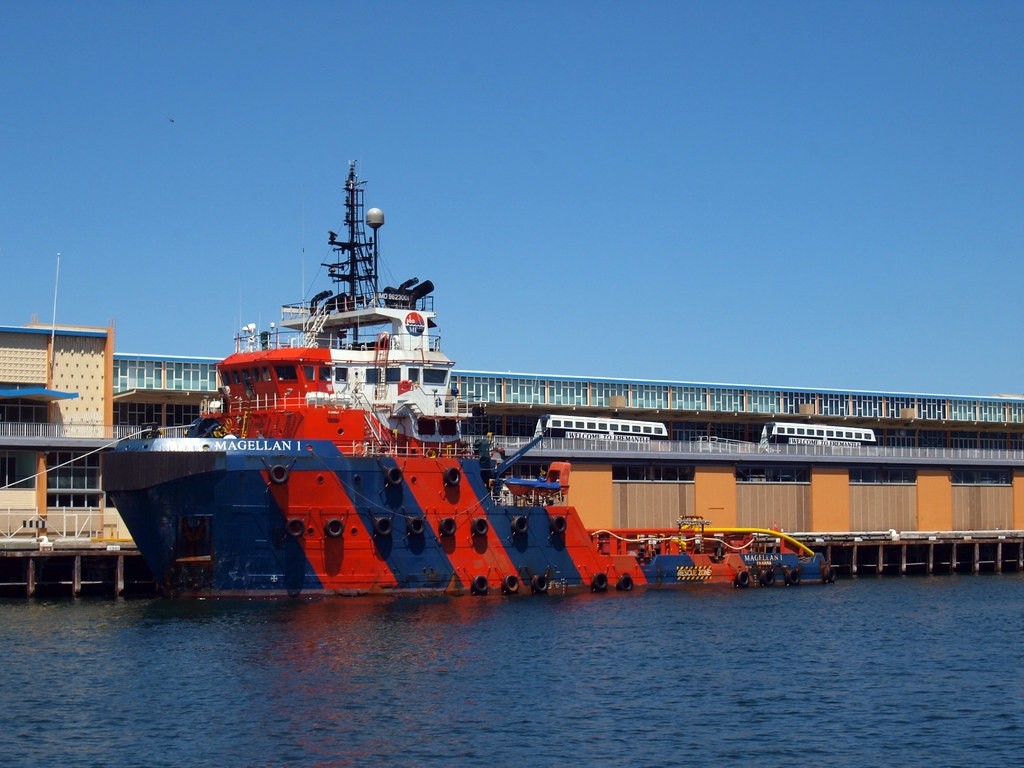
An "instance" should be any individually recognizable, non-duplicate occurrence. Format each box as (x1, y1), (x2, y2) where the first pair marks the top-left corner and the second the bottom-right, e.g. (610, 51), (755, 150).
(591, 572), (607, 591)
(268, 465), (289, 485)
(471, 517), (489, 538)
(285, 518), (306, 538)
(502, 575), (519, 593)
(472, 575), (488, 595)
(399, 380), (411, 393)
(354, 443), (366, 456)
(551, 515), (567, 534)
(616, 574), (633, 591)
(438, 518), (457, 536)
(373, 517), (394, 537)
(734, 571), (749, 588)
(511, 515), (529, 536)
(760, 570), (776, 587)
(325, 518), (344, 538)
(823, 567), (836, 583)
(785, 570), (800, 585)
(531, 575), (549, 594)
(443, 467), (461, 487)
(386, 467), (404, 487)
(408, 516), (425, 536)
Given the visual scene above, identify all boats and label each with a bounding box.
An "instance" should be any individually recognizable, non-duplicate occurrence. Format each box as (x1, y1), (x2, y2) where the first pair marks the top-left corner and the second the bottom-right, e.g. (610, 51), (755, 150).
(330, 393), (351, 405)
(304, 391), (330, 405)
(98, 157), (832, 602)
